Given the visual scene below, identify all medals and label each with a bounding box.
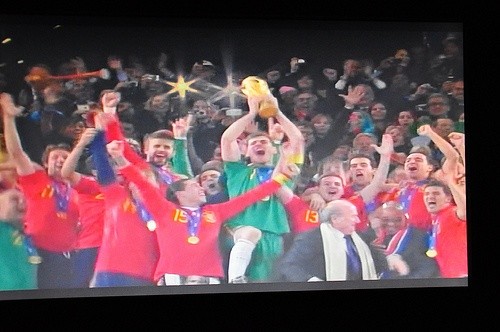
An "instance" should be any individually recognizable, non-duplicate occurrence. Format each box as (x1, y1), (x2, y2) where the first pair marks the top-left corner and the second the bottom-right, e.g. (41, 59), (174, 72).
(146, 219), (158, 232)
(403, 212), (410, 220)
(56, 210), (67, 220)
(262, 193), (271, 202)
(187, 235), (200, 245)
(28, 255), (42, 265)
(426, 248), (438, 258)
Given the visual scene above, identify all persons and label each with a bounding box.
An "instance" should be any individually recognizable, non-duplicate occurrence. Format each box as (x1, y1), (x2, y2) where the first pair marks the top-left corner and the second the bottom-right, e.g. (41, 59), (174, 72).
(0, 91), (77, 288)
(0, 30), (466, 193)
(220, 95), (306, 284)
(270, 134), (393, 237)
(104, 139), (300, 283)
(88, 112), (164, 287)
(60, 125), (107, 287)
(199, 167), (224, 197)
(364, 202), (440, 278)
(421, 164), (468, 278)
(378, 125), (459, 228)
(301, 150), (378, 213)
(0, 187), (39, 291)
(281, 199), (403, 282)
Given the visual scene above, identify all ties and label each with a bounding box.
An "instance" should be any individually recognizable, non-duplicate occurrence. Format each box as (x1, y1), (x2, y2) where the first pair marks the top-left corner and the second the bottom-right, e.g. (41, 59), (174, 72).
(345, 234), (360, 274)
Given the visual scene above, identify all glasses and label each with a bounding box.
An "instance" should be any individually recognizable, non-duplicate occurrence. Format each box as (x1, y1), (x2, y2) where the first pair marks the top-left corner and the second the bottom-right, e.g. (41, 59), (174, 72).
(427, 101), (450, 107)
